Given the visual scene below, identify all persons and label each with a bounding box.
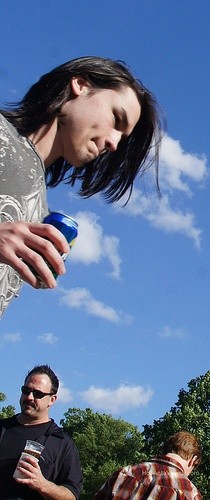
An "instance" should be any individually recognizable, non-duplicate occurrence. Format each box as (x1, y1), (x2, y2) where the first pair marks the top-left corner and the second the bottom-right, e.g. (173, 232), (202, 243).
(1, 56), (166, 320)
(0, 364), (82, 500)
(93, 431), (204, 500)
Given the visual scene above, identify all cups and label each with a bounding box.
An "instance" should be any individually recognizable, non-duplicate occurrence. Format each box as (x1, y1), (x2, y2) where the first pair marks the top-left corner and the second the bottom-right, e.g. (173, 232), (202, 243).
(13, 453), (39, 479)
(24, 440), (45, 460)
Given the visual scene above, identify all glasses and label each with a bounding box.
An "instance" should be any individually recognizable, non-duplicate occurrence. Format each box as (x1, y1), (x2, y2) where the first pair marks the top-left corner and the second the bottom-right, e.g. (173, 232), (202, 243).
(21, 385), (53, 399)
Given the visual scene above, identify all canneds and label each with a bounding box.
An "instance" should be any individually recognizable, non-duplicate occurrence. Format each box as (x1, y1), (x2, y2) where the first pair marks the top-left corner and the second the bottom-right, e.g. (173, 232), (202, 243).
(23, 212), (78, 289)
